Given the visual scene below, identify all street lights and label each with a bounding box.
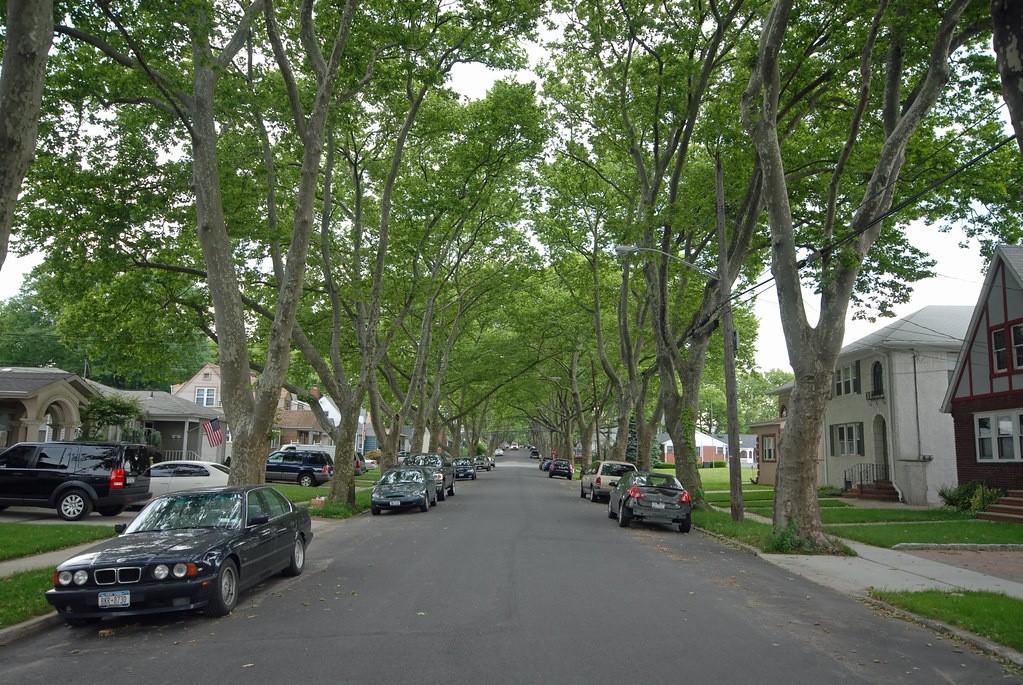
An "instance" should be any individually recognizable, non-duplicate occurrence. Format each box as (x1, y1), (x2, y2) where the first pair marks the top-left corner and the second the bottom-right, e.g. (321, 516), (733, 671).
(614, 244), (744, 523)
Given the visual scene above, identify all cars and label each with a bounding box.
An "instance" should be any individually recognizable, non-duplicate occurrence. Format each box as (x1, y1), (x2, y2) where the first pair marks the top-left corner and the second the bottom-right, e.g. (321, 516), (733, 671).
(124, 461), (230, 510)
(474, 455), (495, 472)
(371, 466), (437, 515)
(539, 458), (555, 471)
(43, 484), (313, 628)
(365, 459), (377, 472)
(397, 451), (411, 463)
(452, 457), (477, 481)
(608, 473), (693, 534)
(494, 441), (539, 459)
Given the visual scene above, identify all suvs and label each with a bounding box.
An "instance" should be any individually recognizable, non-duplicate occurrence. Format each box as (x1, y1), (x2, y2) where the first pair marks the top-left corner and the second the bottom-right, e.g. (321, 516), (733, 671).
(549, 458), (573, 480)
(580, 460), (638, 502)
(0, 441), (154, 521)
(354, 451), (367, 476)
(406, 451), (456, 502)
(264, 450), (334, 487)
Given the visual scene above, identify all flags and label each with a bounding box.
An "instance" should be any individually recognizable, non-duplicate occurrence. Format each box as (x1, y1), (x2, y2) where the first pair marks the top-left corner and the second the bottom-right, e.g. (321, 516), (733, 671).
(202, 417), (223, 448)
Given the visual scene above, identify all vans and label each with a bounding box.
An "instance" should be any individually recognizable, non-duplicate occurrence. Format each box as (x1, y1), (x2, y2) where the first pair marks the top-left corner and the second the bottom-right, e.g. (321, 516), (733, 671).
(276, 445), (336, 463)
